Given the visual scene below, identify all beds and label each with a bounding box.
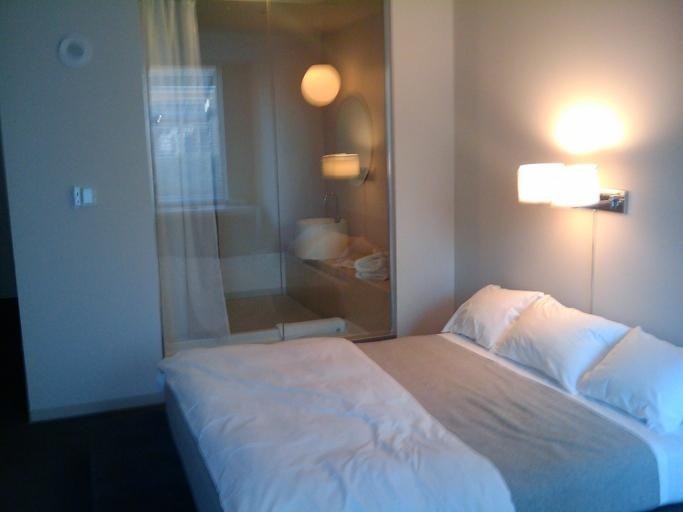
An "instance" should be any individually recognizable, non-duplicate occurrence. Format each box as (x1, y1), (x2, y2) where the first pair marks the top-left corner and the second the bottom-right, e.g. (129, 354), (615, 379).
(162, 333), (683, 512)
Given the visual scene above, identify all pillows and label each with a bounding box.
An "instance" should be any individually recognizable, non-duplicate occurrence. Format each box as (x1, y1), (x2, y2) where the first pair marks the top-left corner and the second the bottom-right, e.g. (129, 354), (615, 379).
(444, 282), (683, 437)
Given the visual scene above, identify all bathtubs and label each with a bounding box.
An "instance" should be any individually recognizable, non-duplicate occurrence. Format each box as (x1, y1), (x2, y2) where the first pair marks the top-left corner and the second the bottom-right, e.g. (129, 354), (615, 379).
(219, 250), (298, 298)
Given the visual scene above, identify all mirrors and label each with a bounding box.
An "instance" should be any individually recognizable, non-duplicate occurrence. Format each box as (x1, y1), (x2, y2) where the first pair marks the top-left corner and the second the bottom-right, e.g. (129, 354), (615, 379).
(332, 88), (375, 187)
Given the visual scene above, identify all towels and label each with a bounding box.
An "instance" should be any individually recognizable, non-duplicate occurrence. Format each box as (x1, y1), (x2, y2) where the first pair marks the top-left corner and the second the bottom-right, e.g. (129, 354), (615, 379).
(353, 251), (389, 283)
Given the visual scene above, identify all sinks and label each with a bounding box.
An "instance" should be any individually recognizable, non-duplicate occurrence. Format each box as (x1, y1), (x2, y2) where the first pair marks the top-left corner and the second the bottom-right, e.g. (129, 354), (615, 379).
(293, 218), (347, 259)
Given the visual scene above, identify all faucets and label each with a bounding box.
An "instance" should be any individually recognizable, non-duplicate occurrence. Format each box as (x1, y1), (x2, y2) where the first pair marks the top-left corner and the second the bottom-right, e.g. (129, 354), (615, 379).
(321, 192), (340, 222)
